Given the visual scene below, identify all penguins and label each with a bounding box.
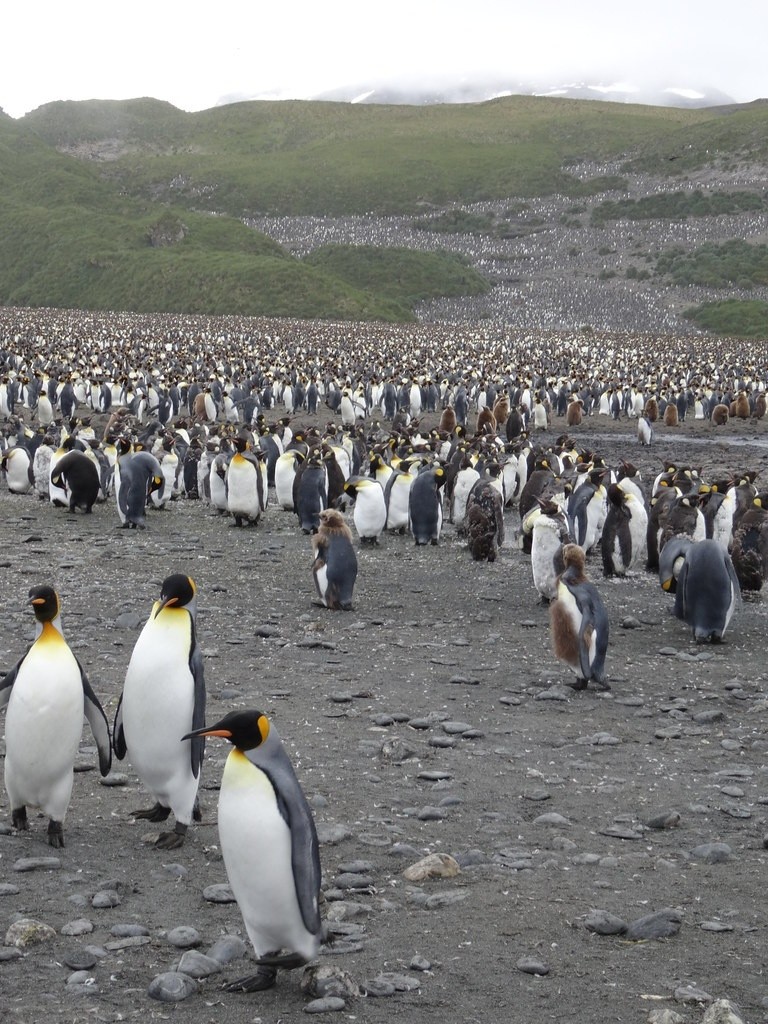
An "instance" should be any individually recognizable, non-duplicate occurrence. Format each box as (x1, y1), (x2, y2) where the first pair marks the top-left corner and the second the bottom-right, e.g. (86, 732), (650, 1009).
(0, 568), (327, 992)
(0, 304), (766, 680)
